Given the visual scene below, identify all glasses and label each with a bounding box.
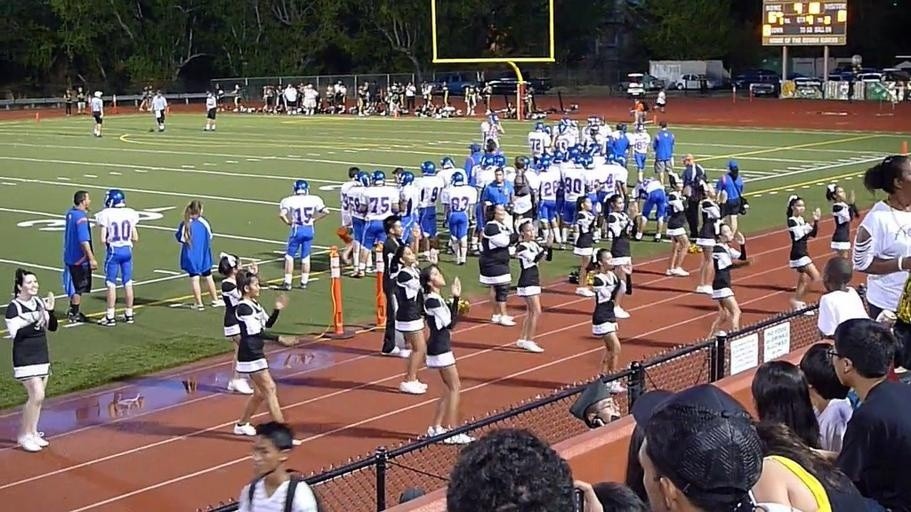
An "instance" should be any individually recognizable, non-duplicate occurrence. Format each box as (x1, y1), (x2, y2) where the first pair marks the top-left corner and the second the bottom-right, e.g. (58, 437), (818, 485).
(825, 346), (846, 360)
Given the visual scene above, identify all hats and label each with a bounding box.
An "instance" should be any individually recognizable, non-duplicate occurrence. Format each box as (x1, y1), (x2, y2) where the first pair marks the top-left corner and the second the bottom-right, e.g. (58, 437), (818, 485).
(728, 159), (739, 170)
(568, 377), (614, 420)
(630, 383), (764, 505)
(467, 145), (481, 152)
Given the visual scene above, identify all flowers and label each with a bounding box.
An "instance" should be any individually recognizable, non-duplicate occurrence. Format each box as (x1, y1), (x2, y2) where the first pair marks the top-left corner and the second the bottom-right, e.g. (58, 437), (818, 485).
(443, 297), (470, 316)
(586, 269), (598, 284)
(688, 243), (697, 254)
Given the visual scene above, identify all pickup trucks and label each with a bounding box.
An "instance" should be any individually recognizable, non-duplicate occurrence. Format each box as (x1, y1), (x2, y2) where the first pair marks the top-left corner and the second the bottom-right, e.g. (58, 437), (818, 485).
(420, 73), (482, 95)
(484, 71), (552, 97)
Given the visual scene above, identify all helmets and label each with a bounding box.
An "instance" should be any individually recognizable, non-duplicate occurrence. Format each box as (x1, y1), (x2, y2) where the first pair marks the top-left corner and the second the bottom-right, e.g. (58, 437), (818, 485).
(479, 154), (495, 171)
(493, 155), (505, 169)
(536, 152), (551, 172)
(604, 152), (614, 162)
(514, 155), (530, 172)
(635, 122), (648, 133)
(439, 156), (457, 168)
(566, 142), (593, 166)
(589, 143), (602, 153)
(451, 172), (464, 186)
(616, 122), (628, 133)
(557, 116), (579, 134)
(370, 170), (385, 186)
(353, 171), (370, 187)
(585, 114), (606, 135)
(103, 189), (126, 207)
(400, 171), (415, 187)
(293, 180), (310, 194)
(553, 149), (565, 163)
(420, 160), (436, 177)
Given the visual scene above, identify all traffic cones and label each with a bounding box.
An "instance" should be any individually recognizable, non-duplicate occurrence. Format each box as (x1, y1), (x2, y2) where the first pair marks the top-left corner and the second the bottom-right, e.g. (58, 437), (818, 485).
(899, 141), (908, 158)
(33, 111), (40, 126)
(393, 107), (400, 119)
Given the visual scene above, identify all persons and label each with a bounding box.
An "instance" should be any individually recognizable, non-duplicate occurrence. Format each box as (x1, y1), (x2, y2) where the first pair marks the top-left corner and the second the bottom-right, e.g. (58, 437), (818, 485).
(847, 80), (856, 102)
(138, 86), (167, 132)
(215, 81), (537, 118)
(218, 253), (302, 444)
(447, 428), (605, 511)
(786, 155), (911, 339)
(594, 319), (910, 510)
(4, 268), (58, 451)
(63, 87), (103, 136)
(62, 190), (139, 326)
(274, 180), (329, 291)
(341, 116), (748, 444)
(204, 91), (217, 131)
(239, 422), (318, 512)
(176, 200), (225, 310)
(631, 87), (668, 125)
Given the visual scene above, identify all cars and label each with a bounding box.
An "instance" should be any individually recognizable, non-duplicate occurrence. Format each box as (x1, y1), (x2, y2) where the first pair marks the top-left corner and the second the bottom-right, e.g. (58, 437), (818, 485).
(615, 58), (910, 101)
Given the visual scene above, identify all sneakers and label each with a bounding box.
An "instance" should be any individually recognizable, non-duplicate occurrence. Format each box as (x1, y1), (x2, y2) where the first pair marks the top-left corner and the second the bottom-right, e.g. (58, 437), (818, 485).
(399, 379), (428, 394)
(211, 299), (226, 308)
(227, 378), (255, 394)
(425, 424), (448, 443)
(273, 279), (292, 291)
(17, 433), (42, 452)
(516, 339), (526, 348)
(701, 285), (714, 294)
(523, 340), (544, 353)
(689, 242), (705, 254)
(613, 304), (631, 319)
(604, 379), (628, 394)
(381, 345), (413, 358)
(349, 264), (375, 277)
(33, 432), (50, 447)
(190, 302), (205, 311)
(635, 231), (644, 241)
(117, 311), (134, 324)
(233, 422), (257, 436)
(444, 427), (476, 444)
(300, 280), (309, 289)
(695, 285), (704, 293)
(672, 267), (690, 276)
(574, 286), (596, 297)
(654, 232), (662, 243)
(97, 314), (116, 326)
(665, 269), (673, 275)
(66, 312), (91, 324)
(491, 314), (514, 323)
(498, 315), (517, 326)
(790, 300), (816, 316)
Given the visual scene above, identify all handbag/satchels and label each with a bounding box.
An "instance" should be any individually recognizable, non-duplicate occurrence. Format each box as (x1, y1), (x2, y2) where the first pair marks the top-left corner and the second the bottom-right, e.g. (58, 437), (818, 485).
(740, 197), (748, 215)
